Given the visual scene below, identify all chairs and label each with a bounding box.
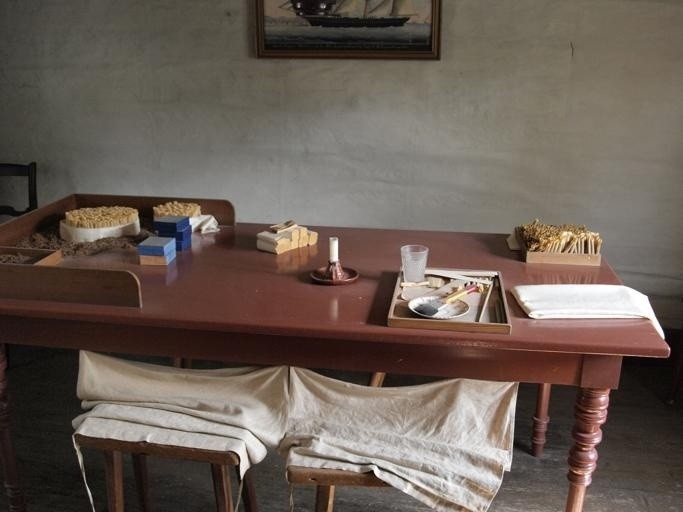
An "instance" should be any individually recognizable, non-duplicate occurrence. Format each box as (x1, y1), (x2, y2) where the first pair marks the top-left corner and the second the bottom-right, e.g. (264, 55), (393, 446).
(0, 158), (40, 223)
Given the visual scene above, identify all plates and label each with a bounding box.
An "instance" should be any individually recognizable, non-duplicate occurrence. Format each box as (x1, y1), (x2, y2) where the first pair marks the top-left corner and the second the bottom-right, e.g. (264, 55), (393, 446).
(407, 296), (470, 319)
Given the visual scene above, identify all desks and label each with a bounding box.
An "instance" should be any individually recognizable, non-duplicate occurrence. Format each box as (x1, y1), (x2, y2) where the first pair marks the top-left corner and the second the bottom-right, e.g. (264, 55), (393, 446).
(0, 186), (671, 511)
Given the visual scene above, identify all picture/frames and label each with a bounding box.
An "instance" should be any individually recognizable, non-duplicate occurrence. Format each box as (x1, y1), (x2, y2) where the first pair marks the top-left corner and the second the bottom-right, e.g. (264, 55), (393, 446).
(248, 0), (441, 64)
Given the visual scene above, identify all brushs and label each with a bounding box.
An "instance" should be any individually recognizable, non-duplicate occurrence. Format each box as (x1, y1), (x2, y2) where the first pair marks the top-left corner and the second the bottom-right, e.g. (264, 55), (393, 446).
(414, 284), (477, 316)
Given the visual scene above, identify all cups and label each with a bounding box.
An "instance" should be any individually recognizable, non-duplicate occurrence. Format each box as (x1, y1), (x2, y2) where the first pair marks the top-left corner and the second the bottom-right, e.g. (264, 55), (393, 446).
(399, 244), (429, 283)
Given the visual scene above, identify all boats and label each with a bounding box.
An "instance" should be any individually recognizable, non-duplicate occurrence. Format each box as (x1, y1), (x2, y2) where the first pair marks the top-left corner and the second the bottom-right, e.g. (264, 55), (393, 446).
(305, 15), (409, 27)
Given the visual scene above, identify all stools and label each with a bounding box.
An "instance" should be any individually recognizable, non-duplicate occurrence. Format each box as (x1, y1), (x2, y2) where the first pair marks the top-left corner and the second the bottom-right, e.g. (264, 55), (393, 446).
(75, 403), (266, 511)
(288, 403), (478, 512)
